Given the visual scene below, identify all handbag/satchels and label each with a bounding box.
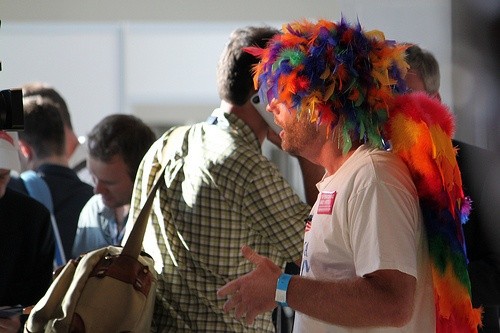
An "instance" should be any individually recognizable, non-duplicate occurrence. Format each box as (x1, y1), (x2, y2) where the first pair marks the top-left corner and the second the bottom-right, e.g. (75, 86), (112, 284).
(24, 244), (159, 333)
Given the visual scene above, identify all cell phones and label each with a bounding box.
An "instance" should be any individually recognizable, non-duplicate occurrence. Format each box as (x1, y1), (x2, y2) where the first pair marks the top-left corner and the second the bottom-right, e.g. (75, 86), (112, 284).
(250, 92), (283, 135)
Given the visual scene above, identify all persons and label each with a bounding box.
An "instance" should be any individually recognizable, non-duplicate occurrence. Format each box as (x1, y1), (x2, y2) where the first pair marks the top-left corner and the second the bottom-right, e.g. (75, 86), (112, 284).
(72, 113), (158, 258)
(216, 19), (437, 333)
(401, 44), (500, 332)
(0, 83), (96, 333)
(131, 29), (331, 333)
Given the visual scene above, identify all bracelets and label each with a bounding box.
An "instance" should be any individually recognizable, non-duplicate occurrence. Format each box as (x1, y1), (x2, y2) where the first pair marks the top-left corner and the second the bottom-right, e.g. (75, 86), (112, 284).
(276, 274), (292, 307)
